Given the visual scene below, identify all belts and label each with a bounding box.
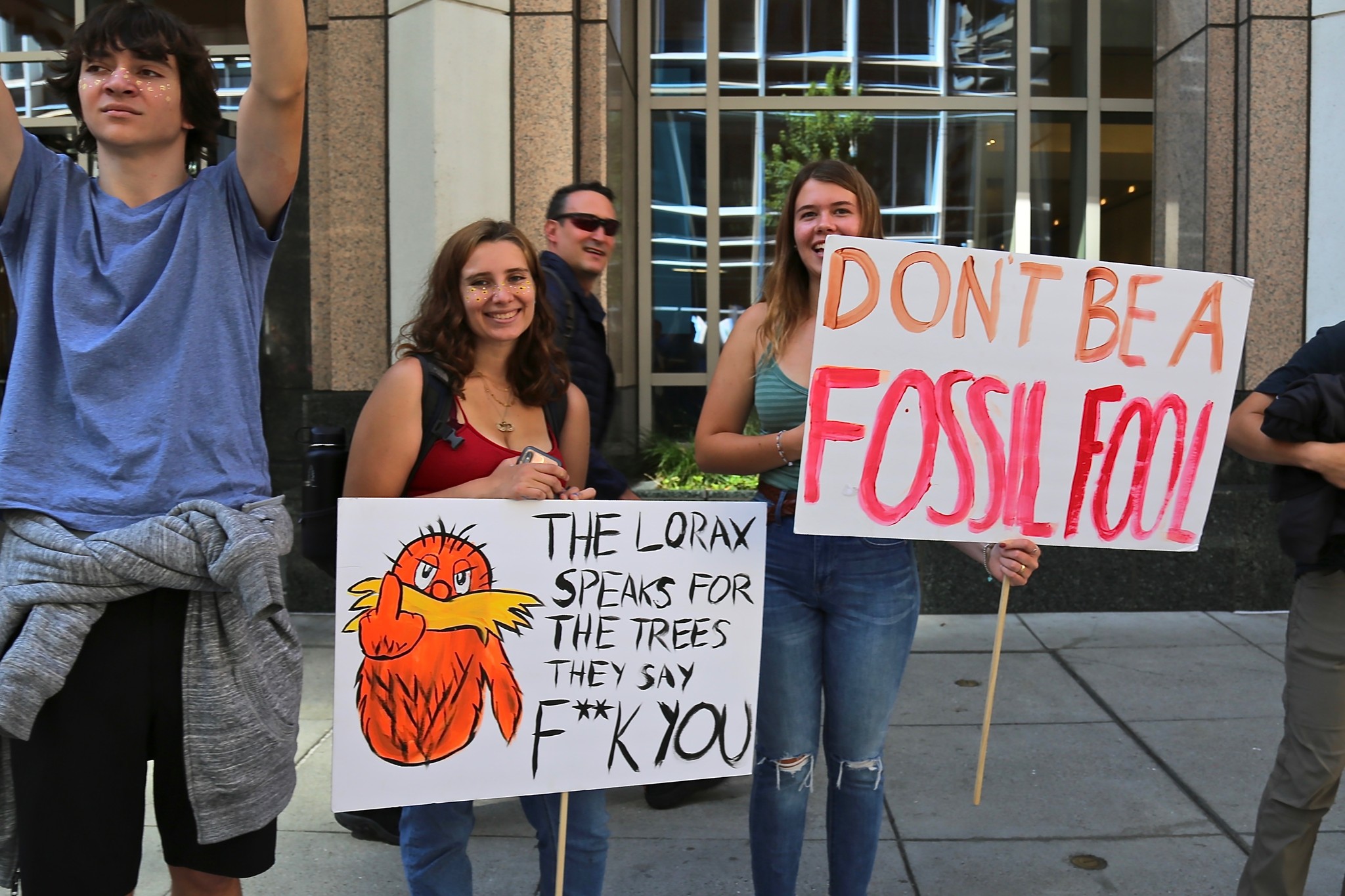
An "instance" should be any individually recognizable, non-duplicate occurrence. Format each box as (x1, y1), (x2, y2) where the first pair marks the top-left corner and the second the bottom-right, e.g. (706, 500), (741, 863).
(755, 481), (797, 524)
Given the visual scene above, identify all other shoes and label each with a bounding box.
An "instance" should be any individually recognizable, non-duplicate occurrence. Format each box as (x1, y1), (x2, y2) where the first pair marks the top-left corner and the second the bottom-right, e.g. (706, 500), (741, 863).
(334, 806), (401, 846)
(645, 775), (729, 810)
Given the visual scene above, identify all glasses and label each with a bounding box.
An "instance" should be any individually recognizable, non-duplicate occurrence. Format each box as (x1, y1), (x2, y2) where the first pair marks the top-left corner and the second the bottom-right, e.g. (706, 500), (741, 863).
(553, 212), (622, 237)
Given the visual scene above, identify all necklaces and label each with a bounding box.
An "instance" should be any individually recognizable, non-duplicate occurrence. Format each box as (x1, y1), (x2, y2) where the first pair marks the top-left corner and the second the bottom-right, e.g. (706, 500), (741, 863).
(476, 366), (518, 432)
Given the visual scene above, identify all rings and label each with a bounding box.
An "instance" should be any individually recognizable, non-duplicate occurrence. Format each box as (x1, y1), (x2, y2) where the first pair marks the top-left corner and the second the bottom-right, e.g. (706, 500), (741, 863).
(1030, 545), (1039, 554)
(1017, 565), (1025, 574)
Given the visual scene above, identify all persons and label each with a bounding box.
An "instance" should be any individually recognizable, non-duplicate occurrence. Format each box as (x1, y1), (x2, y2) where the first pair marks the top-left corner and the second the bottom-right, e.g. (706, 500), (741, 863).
(0, 0), (309, 896)
(694, 161), (1041, 896)
(331, 181), (731, 842)
(1230, 320), (1345, 896)
(342, 218), (611, 896)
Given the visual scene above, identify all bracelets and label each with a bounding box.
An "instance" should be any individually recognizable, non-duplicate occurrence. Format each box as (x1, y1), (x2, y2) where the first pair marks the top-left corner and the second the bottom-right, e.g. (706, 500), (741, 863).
(983, 544), (990, 574)
(776, 430), (793, 465)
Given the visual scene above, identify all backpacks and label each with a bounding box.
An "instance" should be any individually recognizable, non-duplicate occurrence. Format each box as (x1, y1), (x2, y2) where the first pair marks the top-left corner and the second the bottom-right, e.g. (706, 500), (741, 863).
(299, 343), (568, 580)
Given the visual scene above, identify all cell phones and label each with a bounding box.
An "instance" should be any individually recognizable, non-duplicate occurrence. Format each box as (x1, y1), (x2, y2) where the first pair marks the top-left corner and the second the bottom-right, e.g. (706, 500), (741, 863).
(515, 446), (562, 468)
